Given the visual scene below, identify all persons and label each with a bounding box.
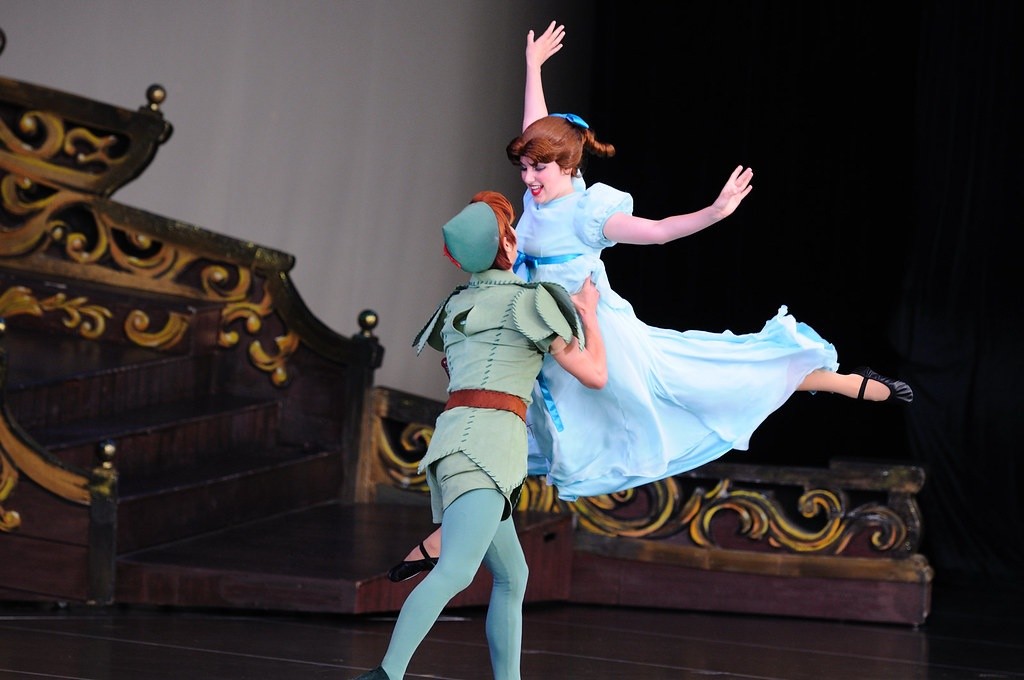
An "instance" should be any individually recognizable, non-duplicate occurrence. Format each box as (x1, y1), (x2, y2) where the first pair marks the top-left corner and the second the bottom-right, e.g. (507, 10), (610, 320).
(506, 21), (913, 502)
(355, 191), (607, 680)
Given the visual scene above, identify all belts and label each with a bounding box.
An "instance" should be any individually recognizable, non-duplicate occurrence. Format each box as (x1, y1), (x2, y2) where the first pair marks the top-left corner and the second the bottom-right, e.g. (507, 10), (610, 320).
(443, 389), (529, 424)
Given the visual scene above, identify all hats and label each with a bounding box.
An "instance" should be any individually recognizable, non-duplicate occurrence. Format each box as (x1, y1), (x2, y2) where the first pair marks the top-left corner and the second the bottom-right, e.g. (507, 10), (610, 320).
(442, 202), (500, 273)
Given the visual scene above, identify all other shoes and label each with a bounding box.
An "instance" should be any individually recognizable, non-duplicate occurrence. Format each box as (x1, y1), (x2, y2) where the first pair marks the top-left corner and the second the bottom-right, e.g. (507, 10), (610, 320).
(848, 363), (913, 408)
(389, 542), (442, 583)
(354, 666), (389, 680)
